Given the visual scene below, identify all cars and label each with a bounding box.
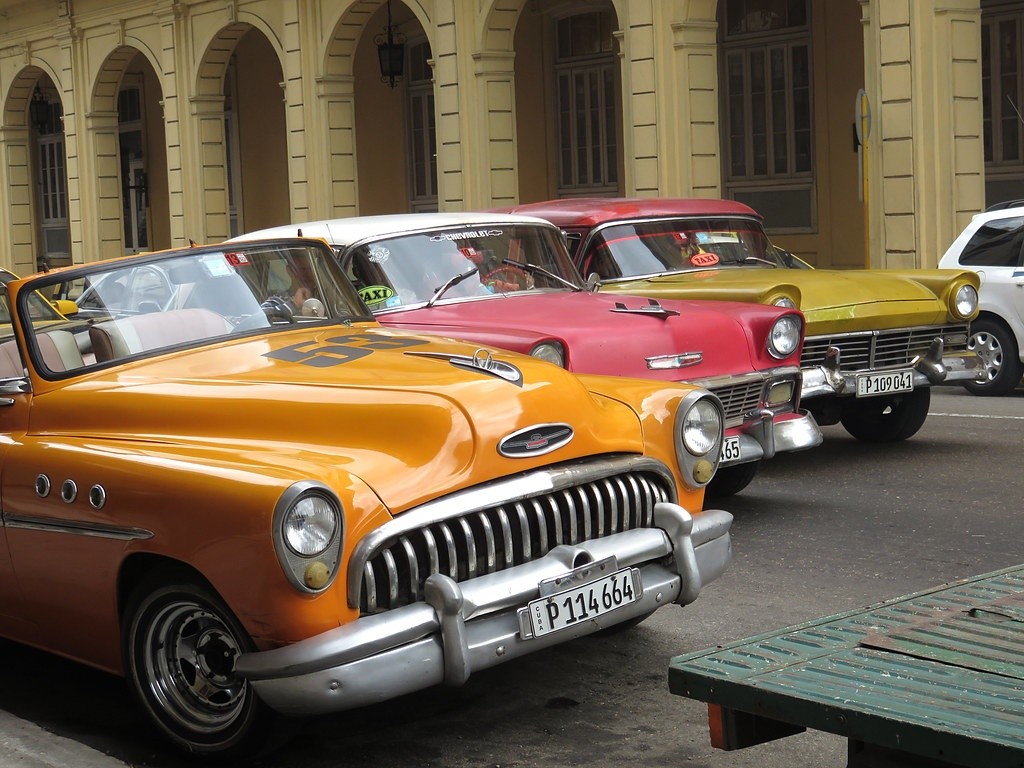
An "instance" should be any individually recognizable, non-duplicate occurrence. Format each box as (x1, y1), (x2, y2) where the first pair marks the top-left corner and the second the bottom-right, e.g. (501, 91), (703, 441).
(2, 233), (738, 758)
(927, 205), (1024, 392)
(217, 214), (825, 500)
(453, 197), (991, 451)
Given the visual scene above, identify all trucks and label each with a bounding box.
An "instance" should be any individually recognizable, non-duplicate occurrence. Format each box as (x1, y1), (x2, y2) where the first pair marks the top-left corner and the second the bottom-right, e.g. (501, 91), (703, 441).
(663, 550), (1023, 768)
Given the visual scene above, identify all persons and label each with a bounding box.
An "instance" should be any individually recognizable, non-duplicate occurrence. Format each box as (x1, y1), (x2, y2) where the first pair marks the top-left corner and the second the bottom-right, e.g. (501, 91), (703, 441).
(258, 249), (324, 314)
(448, 247), (535, 295)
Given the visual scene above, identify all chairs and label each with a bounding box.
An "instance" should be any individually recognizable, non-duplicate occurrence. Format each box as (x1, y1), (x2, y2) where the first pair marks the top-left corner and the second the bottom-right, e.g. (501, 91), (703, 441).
(88, 308), (237, 363)
(74, 329), (97, 366)
(0, 329), (86, 380)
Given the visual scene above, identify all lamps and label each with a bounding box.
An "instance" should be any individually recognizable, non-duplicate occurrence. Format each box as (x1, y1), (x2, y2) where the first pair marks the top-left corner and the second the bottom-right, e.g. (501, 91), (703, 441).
(373, 0), (408, 91)
(30, 81), (49, 135)
